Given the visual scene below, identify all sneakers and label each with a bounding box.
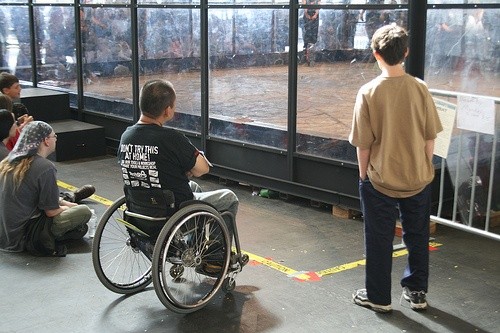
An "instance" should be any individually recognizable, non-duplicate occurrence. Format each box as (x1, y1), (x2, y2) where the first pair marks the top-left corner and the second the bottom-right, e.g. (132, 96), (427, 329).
(352, 288), (391, 313)
(403, 286), (428, 311)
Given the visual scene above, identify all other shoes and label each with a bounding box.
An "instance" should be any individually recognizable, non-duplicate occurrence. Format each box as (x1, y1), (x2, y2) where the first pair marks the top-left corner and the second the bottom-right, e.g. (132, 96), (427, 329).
(205, 253), (249, 273)
(64, 223), (88, 240)
(74, 185), (96, 202)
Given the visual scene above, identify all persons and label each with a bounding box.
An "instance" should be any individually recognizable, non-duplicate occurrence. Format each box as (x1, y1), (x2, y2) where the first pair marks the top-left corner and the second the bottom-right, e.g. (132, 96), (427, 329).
(118, 79), (249, 272)
(349, 25), (443, 313)
(0, 109), (96, 204)
(0, 0), (290, 81)
(0, 120), (92, 256)
(300, 0), (321, 66)
(425, 7), (500, 72)
(323, 0), (409, 64)
(0, 71), (33, 151)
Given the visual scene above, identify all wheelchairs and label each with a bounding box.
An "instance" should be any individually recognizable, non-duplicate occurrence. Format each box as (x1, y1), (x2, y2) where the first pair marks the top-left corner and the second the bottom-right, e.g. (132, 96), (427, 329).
(91, 189), (249, 315)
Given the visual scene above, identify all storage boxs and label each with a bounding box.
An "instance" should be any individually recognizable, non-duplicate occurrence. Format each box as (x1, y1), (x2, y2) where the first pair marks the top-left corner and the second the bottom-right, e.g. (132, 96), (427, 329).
(332, 205), (360, 219)
(395, 220), (436, 237)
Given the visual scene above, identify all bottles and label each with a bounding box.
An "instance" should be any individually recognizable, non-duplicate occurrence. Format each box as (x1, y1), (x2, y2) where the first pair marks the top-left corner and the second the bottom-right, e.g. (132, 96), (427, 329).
(88, 209), (97, 238)
